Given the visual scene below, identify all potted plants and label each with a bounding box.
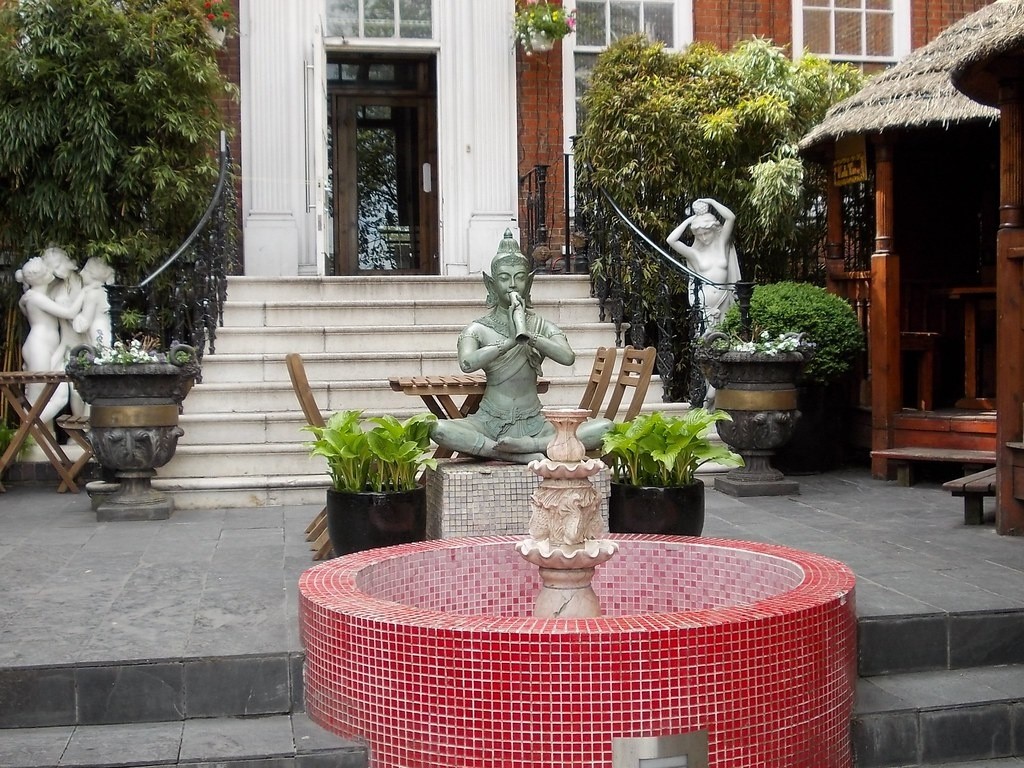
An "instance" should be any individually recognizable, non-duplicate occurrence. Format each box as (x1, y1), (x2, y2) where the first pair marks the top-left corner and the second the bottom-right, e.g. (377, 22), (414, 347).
(298, 408), (439, 557)
(600, 407), (746, 537)
(747, 281), (865, 478)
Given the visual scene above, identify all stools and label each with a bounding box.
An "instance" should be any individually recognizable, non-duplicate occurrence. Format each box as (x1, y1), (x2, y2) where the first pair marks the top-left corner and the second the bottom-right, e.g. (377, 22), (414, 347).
(900, 332), (941, 411)
(57, 416), (97, 494)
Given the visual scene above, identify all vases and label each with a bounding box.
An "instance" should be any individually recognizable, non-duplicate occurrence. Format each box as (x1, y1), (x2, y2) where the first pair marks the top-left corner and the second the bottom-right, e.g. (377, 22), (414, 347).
(696, 328), (817, 498)
(530, 32), (555, 51)
(211, 26), (227, 46)
(66, 342), (203, 523)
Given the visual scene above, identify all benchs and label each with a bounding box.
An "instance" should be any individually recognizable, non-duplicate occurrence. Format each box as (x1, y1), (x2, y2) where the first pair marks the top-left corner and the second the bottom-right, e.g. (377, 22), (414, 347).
(388, 372), (550, 486)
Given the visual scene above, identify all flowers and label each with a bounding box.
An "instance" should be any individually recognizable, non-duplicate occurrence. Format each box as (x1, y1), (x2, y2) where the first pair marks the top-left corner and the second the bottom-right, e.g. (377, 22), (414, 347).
(62, 327), (200, 370)
(697, 329), (817, 357)
(508, 0), (577, 56)
(198, 0), (237, 31)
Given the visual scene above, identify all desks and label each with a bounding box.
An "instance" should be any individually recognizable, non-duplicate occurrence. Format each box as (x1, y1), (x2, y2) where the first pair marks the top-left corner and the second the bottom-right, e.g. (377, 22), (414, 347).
(937, 287), (997, 411)
(0, 371), (81, 494)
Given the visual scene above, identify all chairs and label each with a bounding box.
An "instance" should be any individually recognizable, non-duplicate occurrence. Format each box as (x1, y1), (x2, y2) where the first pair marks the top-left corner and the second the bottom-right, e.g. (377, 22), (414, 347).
(285, 352), (343, 561)
(578, 346), (657, 468)
